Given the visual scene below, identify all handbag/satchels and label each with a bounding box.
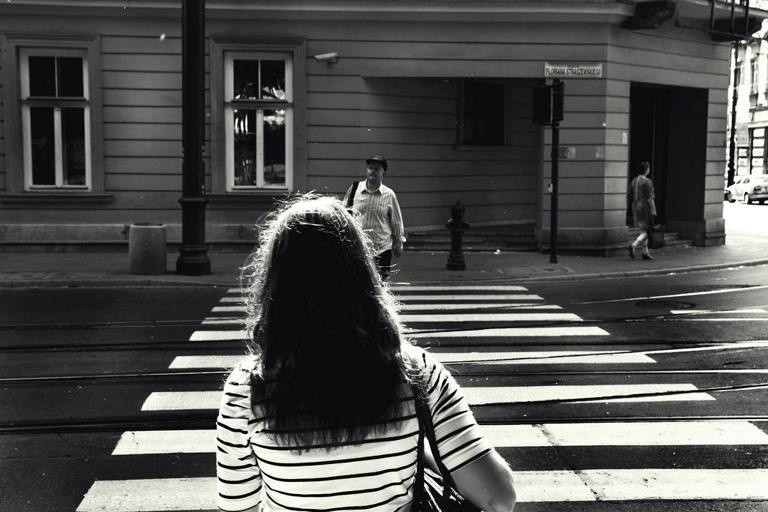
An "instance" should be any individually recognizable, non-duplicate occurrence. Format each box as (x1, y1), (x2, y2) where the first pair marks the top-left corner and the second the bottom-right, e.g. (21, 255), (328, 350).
(412, 467), (482, 512)
(646, 225), (665, 248)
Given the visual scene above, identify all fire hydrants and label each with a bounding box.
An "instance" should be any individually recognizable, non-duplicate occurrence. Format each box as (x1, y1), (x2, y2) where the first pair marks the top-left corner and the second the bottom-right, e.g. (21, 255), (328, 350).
(446, 200), (470, 270)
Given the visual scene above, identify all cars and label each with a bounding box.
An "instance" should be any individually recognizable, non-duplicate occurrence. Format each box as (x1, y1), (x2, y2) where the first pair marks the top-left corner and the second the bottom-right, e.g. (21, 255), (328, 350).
(725, 173), (768, 205)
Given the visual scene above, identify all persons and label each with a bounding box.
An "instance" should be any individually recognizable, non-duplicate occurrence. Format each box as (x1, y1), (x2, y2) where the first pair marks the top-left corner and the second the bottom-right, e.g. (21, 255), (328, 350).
(215, 187), (517, 512)
(627, 161), (658, 260)
(342, 154), (407, 284)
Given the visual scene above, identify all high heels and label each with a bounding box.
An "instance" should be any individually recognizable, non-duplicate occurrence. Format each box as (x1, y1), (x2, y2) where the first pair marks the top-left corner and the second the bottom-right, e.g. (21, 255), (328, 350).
(628, 245), (654, 260)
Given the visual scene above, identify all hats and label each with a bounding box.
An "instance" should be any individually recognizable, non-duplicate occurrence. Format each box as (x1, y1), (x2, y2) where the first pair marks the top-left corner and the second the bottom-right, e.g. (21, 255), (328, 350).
(365, 155), (387, 171)
